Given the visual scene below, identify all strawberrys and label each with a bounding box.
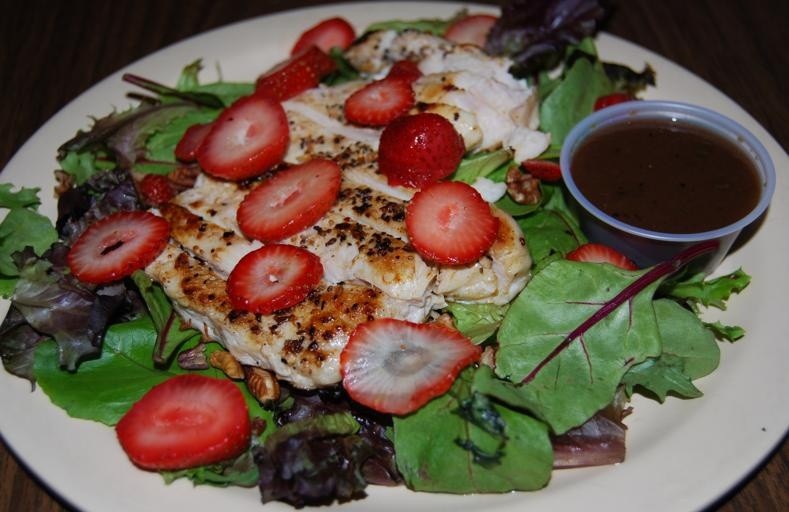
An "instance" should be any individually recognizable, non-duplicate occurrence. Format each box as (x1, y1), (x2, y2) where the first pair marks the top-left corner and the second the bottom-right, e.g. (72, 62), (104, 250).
(69, 14), (638, 471)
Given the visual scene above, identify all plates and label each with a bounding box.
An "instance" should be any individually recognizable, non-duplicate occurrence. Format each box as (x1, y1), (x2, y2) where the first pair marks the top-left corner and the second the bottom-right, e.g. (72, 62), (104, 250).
(0, 0), (789, 512)
